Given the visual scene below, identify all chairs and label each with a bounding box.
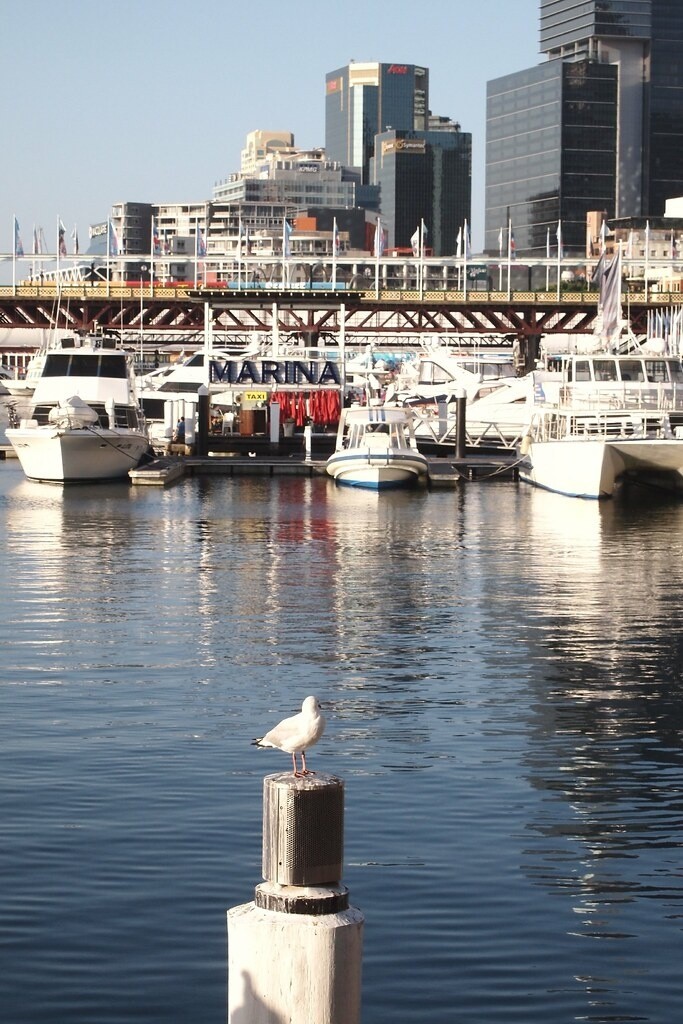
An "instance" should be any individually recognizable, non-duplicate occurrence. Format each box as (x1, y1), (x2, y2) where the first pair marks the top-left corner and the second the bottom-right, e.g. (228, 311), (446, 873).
(222, 412), (234, 436)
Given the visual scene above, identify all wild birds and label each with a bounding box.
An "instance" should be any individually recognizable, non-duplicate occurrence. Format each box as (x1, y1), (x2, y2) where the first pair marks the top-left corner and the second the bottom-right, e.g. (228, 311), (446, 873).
(250, 695), (326, 781)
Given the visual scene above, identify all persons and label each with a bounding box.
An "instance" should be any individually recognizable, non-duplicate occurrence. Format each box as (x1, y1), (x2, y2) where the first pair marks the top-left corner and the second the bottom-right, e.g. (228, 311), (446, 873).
(344, 386), (366, 408)
(174, 417), (185, 443)
(212, 395), (242, 432)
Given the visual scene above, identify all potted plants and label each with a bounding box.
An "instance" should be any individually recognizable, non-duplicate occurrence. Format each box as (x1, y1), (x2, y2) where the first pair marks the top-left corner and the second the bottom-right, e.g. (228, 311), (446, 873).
(283, 418), (295, 438)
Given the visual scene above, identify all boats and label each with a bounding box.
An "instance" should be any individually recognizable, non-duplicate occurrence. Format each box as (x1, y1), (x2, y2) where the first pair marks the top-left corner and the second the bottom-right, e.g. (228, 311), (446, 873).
(5, 284), (149, 484)
(324, 371), (429, 492)
(0, 349), (47, 396)
(135, 286), (683, 501)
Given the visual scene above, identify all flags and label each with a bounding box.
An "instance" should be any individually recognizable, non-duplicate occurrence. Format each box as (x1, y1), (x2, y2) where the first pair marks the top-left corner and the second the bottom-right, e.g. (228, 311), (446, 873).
(375, 225), (384, 257)
(197, 225), (207, 272)
(556, 224), (610, 260)
(423, 223), (428, 257)
(335, 224), (340, 259)
(625, 225), (677, 259)
(591, 252), (681, 352)
(410, 231), (417, 268)
(59, 219), (67, 256)
(285, 220), (292, 257)
(498, 225), (515, 258)
(15, 218), (24, 258)
(236, 223), (251, 262)
(153, 223), (162, 256)
(34, 227), (38, 253)
(71, 228), (78, 253)
(164, 230), (169, 255)
(110, 222), (118, 256)
(456, 224), (472, 259)
